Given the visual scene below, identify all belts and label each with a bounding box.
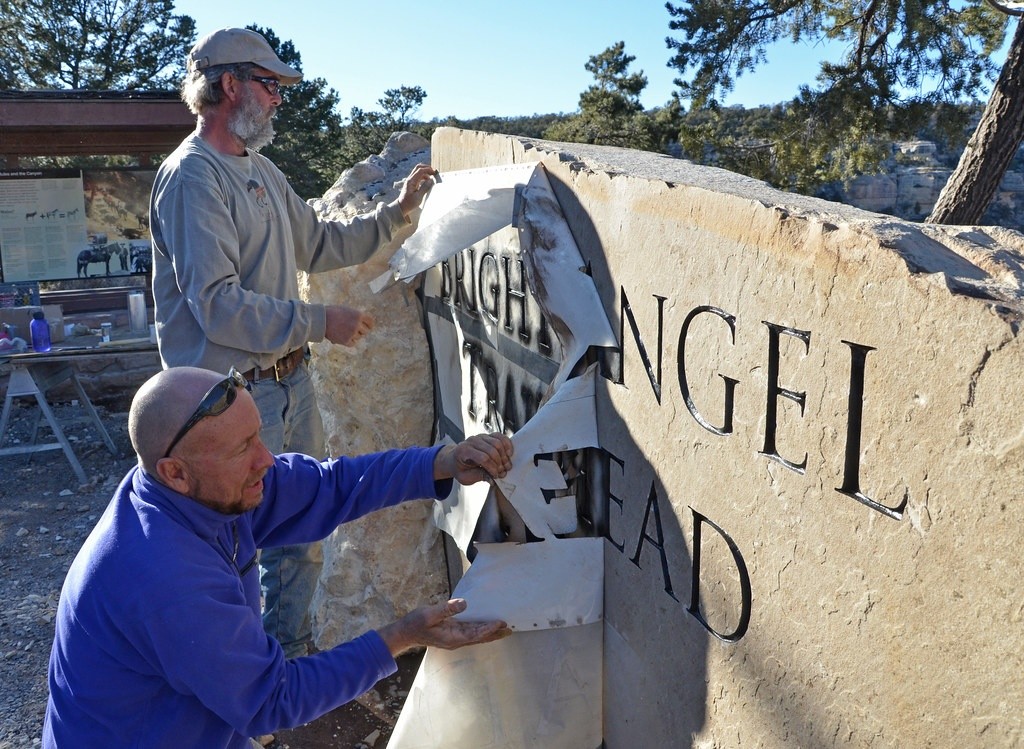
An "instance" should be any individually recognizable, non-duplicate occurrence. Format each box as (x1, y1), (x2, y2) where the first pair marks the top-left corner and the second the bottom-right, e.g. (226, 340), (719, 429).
(242, 343), (310, 382)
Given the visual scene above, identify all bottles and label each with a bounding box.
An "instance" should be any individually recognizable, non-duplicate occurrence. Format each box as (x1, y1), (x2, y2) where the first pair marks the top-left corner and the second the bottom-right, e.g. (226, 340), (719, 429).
(28, 308), (51, 352)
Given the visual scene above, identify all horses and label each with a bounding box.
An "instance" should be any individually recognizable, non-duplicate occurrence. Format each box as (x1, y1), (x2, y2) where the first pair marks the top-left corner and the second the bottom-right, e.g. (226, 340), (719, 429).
(77, 184), (149, 277)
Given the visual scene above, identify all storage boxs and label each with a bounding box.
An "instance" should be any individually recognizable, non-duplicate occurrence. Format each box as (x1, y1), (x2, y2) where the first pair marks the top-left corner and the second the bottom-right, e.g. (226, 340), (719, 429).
(0, 305), (66, 343)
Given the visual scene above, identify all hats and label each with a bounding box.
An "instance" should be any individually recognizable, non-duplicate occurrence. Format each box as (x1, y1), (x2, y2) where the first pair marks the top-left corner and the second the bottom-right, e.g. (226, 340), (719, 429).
(187, 28), (303, 85)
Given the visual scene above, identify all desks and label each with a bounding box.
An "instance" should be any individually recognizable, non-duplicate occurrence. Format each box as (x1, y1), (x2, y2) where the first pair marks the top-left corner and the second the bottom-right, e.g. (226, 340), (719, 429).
(0, 328), (160, 485)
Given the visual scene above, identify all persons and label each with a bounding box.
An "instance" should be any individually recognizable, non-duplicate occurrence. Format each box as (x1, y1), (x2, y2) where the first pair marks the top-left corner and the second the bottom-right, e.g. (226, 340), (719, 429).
(41, 367), (514, 749)
(148, 28), (436, 749)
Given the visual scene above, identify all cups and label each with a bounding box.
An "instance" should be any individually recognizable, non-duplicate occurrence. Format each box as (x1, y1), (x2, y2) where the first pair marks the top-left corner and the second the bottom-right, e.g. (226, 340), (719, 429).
(127, 290), (147, 333)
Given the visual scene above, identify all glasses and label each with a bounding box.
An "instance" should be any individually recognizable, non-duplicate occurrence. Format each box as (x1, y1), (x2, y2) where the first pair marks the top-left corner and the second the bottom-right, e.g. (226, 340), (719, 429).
(162, 364), (249, 458)
(212, 73), (283, 96)
